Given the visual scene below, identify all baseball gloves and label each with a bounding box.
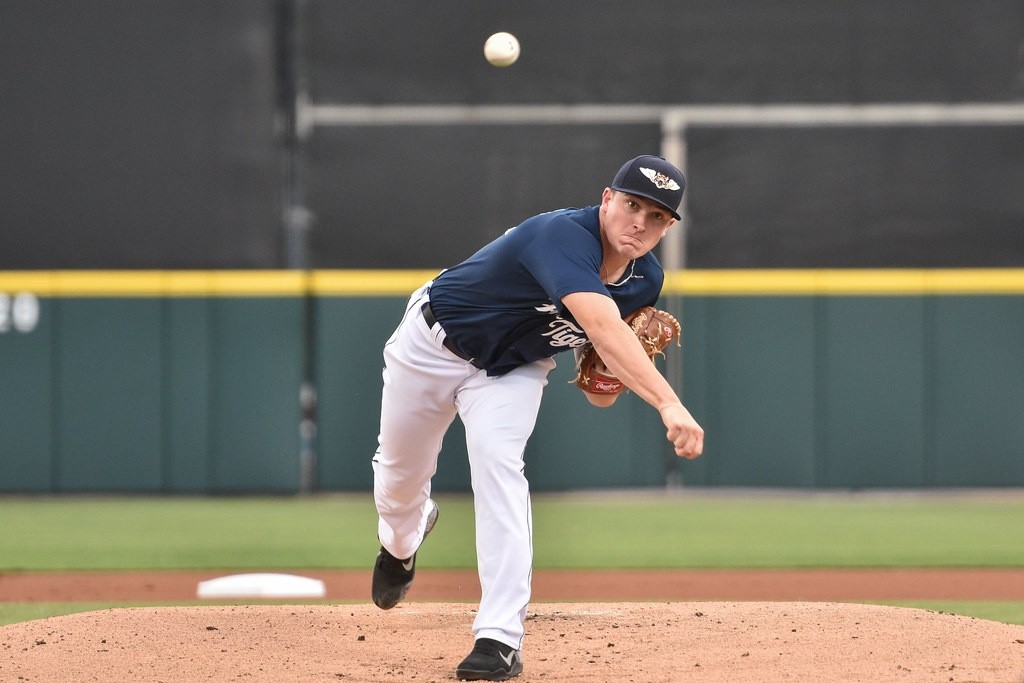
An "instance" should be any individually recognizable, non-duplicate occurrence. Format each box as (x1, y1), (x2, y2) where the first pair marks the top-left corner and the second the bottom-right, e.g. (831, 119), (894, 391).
(575, 305), (681, 394)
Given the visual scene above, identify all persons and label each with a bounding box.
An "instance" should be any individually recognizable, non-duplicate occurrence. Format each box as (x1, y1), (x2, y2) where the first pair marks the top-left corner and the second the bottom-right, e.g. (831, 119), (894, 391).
(371, 154), (704, 682)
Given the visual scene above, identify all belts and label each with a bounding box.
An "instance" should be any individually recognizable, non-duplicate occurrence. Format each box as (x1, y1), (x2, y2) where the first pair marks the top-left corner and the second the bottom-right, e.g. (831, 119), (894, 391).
(421, 286), (485, 371)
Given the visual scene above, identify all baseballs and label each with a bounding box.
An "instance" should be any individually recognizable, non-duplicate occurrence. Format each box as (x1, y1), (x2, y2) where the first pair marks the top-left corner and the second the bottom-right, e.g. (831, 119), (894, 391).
(483, 31), (521, 67)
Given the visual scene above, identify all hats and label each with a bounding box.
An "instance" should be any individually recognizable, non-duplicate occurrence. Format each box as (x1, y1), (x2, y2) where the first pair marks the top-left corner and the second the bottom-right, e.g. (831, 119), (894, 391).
(611, 155), (686, 221)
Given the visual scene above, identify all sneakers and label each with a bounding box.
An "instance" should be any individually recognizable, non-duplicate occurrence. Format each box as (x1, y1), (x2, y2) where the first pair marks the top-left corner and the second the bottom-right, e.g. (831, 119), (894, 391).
(371, 498), (439, 610)
(456, 638), (523, 680)
